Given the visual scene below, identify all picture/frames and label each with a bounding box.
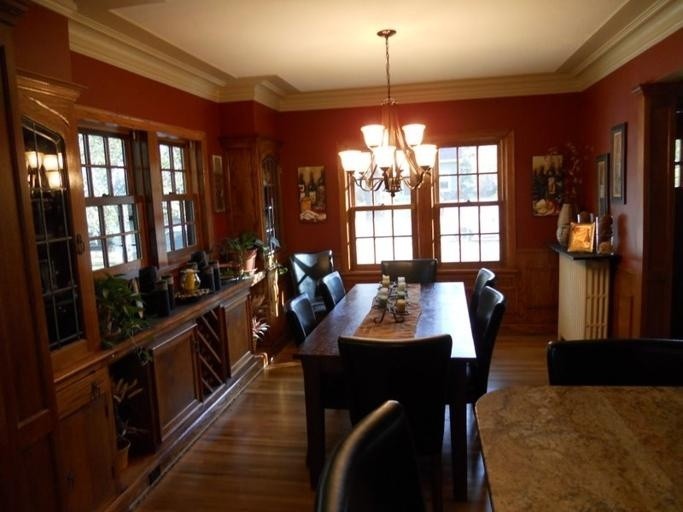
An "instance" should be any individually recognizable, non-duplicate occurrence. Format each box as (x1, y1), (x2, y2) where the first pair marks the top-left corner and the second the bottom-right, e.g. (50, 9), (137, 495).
(595, 152), (611, 216)
(609, 120), (628, 206)
(208, 151), (227, 215)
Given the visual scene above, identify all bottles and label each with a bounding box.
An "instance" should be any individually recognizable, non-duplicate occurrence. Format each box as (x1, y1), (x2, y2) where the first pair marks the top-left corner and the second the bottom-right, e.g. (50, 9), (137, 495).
(128, 251), (222, 320)
(298, 168), (325, 206)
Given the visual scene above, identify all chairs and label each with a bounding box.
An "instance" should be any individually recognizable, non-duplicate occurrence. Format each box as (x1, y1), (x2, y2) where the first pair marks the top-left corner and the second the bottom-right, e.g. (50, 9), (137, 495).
(544, 336), (683, 387)
(313, 398), (428, 512)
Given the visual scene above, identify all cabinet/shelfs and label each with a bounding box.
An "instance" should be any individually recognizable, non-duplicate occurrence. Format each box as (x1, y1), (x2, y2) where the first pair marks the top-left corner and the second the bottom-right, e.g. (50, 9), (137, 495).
(222, 290), (253, 379)
(15, 73), (101, 376)
(256, 250), (292, 337)
(56, 364), (118, 512)
(225, 134), (286, 256)
(150, 322), (204, 444)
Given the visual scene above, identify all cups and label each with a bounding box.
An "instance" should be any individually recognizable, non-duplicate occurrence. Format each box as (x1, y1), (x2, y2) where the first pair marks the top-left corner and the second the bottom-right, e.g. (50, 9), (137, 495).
(299, 199), (310, 214)
(577, 213), (592, 224)
(595, 216), (614, 255)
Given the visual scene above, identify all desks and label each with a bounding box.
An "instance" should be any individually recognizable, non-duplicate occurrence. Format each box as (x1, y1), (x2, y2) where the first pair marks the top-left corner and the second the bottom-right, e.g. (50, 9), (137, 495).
(474, 382), (683, 512)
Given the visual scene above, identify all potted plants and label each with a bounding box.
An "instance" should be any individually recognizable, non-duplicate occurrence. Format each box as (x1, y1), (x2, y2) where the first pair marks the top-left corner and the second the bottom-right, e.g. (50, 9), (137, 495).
(218, 230), (289, 277)
(251, 314), (272, 353)
(111, 377), (150, 471)
(92, 269), (157, 363)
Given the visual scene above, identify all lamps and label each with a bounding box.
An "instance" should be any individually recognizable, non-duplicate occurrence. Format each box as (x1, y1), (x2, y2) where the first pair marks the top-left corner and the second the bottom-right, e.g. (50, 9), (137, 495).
(336, 28), (438, 196)
(23, 145), (66, 196)
